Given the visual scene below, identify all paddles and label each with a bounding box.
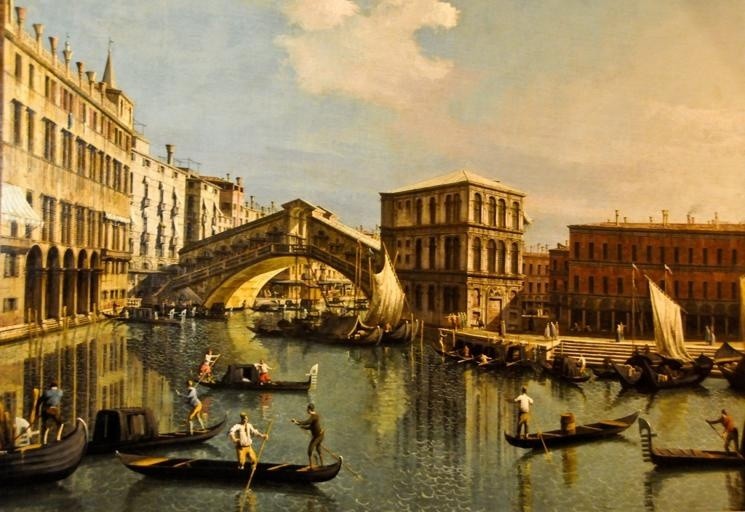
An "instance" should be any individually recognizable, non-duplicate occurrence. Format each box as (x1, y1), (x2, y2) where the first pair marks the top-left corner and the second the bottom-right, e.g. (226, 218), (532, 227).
(705, 419), (745, 466)
(0, 387), (75, 452)
(292, 419), (362, 481)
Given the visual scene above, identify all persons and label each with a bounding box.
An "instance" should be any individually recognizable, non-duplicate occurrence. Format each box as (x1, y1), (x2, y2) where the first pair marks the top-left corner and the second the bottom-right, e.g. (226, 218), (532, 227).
(554, 321), (559, 339)
(442, 310), (469, 332)
(620, 321), (625, 341)
(438, 336), (444, 351)
(578, 353), (588, 376)
(707, 408), (741, 456)
(383, 320), (392, 333)
(506, 385), (534, 438)
(462, 344), (470, 357)
(502, 319), (507, 337)
(615, 323), (620, 343)
(549, 321), (556, 341)
(479, 353), (488, 363)
(704, 326), (711, 343)
(175, 380), (205, 435)
(226, 411), (268, 469)
(498, 320), (504, 337)
(112, 301), (120, 315)
(544, 322), (550, 341)
(295, 403), (327, 470)
(34, 382), (65, 446)
(197, 348), (220, 384)
(251, 358), (274, 384)
